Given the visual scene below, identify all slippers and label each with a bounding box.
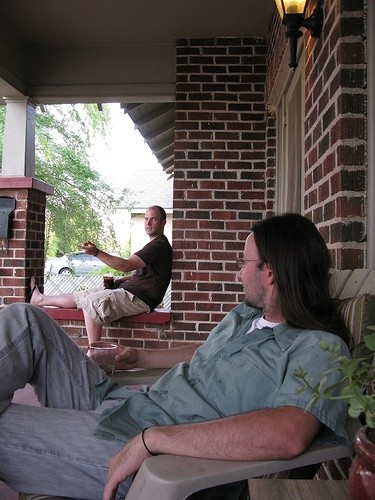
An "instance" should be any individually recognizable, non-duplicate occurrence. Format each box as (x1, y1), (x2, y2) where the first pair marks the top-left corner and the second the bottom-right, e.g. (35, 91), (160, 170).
(26, 280), (37, 303)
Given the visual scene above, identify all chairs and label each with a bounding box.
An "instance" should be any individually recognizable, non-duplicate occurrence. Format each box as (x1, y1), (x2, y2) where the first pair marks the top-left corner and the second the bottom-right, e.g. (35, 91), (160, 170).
(18, 268), (375, 500)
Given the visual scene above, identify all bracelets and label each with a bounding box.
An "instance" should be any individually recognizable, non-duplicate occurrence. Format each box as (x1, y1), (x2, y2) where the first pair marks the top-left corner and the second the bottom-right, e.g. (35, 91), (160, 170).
(142, 428), (158, 456)
(93, 249), (100, 256)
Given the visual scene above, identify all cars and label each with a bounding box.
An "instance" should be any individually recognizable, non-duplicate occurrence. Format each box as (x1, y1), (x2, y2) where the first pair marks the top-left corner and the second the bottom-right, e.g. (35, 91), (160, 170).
(44, 251), (107, 277)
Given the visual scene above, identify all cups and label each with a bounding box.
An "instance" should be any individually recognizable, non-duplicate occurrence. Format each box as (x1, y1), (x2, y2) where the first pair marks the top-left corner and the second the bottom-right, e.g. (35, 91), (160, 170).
(103, 274), (113, 289)
(89, 341), (117, 376)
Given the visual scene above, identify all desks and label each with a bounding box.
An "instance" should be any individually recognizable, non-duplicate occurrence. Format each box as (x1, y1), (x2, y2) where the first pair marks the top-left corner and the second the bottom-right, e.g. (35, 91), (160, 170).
(237, 478), (352, 500)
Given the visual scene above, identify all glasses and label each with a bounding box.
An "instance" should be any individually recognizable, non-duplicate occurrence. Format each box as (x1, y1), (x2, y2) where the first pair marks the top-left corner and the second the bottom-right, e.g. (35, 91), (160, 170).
(236, 256), (260, 271)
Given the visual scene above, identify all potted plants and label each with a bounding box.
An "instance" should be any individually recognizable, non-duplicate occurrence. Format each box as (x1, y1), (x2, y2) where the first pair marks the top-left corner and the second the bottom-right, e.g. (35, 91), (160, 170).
(294, 324), (375, 500)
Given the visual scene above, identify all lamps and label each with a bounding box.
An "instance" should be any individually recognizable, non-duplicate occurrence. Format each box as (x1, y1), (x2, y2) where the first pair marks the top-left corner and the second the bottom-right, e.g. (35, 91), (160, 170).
(275, 0), (324, 72)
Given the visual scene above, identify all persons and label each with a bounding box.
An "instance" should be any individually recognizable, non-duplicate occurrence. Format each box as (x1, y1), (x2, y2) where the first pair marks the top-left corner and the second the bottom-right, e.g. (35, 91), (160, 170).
(27, 205), (172, 358)
(0, 213), (355, 500)
